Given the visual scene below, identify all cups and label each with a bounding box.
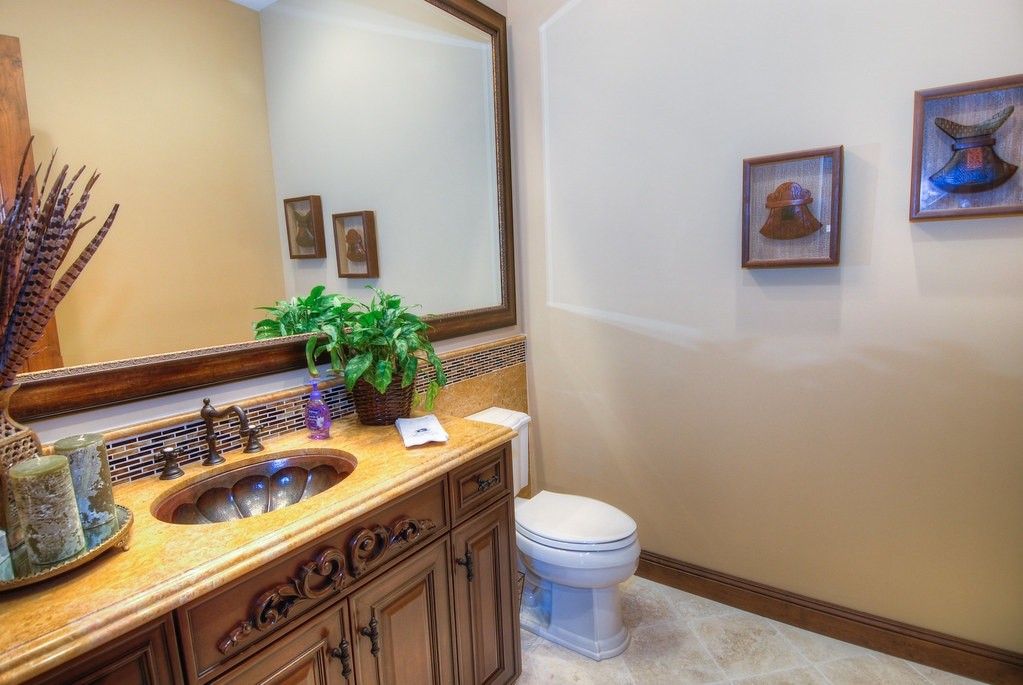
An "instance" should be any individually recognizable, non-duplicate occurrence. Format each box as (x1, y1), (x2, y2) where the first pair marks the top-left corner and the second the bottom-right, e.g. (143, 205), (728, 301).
(54, 433), (117, 527)
(7, 455), (86, 565)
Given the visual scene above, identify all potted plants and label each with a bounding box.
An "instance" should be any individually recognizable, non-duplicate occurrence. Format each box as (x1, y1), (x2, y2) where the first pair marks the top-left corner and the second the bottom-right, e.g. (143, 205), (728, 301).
(251, 285), (345, 340)
(306, 286), (447, 427)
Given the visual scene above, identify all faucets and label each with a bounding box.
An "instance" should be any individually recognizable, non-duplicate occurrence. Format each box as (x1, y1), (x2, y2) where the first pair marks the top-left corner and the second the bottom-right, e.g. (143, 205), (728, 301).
(200, 398), (252, 466)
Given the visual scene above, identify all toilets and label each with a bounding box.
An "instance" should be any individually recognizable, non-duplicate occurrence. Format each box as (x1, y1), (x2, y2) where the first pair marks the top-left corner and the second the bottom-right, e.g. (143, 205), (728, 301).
(461, 406), (640, 663)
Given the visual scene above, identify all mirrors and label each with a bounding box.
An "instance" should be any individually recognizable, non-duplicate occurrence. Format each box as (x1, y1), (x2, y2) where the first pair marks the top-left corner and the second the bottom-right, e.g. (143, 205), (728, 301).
(0, 0), (517, 426)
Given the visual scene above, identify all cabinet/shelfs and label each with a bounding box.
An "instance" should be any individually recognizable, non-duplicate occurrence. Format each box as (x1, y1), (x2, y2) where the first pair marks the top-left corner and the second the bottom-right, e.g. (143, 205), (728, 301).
(21, 440), (523, 685)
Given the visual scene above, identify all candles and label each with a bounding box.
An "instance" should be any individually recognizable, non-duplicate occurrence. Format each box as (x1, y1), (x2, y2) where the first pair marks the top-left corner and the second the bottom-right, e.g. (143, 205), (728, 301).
(53, 434), (116, 530)
(7, 453), (85, 565)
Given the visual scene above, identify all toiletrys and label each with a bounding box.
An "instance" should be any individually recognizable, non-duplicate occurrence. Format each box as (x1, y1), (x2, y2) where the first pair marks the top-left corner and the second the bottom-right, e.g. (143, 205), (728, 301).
(304, 379), (332, 441)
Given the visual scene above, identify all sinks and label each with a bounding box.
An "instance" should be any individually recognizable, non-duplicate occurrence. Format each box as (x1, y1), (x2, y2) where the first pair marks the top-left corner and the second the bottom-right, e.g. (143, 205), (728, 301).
(148, 447), (358, 526)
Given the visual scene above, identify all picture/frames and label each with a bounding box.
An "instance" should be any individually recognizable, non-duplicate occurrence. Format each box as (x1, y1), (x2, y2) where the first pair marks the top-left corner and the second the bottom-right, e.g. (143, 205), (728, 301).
(908, 74), (1023, 222)
(284, 195), (327, 259)
(332, 211), (379, 279)
(741, 144), (844, 269)
